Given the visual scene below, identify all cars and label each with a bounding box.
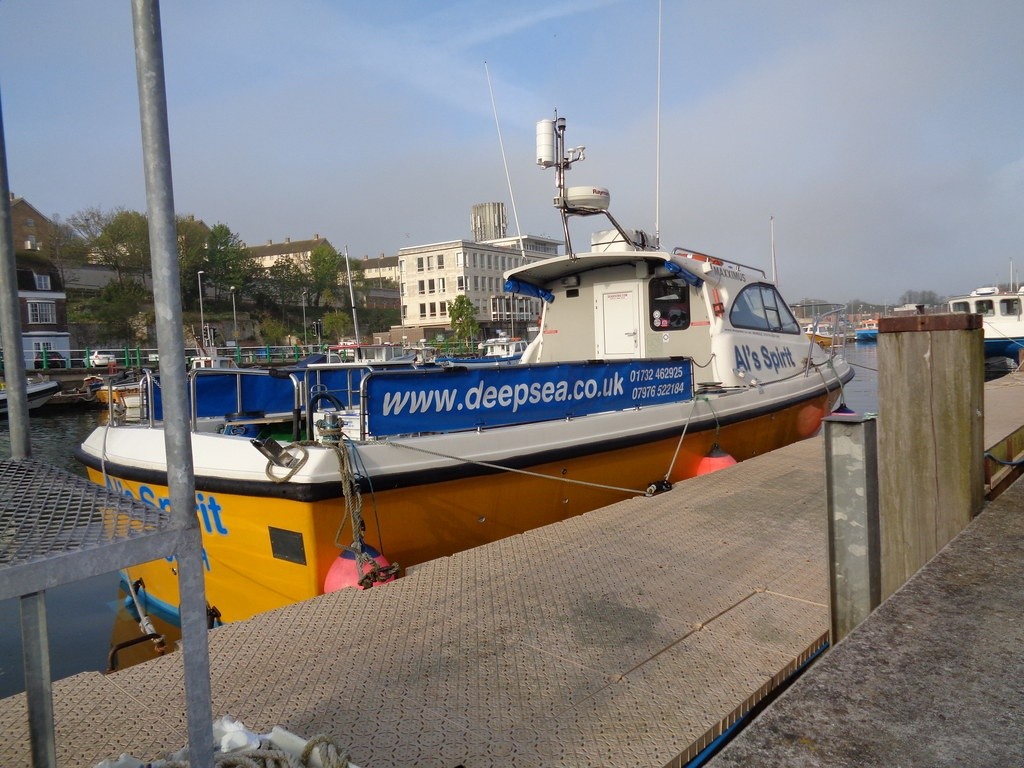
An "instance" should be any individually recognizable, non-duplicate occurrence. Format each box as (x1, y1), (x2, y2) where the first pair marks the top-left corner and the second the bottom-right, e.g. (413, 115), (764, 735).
(35, 351), (66, 369)
(82, 349), (117, 369)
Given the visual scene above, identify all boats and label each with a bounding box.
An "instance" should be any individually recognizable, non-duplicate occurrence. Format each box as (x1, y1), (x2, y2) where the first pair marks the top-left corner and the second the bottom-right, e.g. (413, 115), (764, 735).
(0, 353), (531, 428)
(945, 286), (1024, 382)
(77, 109), (856, 678)
(801, 314), (881, 354)
(482, 340), (529, 358)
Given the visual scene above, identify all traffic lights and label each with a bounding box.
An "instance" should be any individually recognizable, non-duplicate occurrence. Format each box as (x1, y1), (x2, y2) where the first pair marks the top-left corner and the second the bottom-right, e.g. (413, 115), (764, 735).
(213, 327), (218, 339)
(203, 326), (209, 341)
(311, 322), (319, 337)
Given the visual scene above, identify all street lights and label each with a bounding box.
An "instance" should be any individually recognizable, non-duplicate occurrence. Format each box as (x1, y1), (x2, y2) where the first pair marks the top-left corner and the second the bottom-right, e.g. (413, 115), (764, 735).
(197, 271), (209, 359)
(230, 286), (242, 363)
(302, 292), (308, 344)
